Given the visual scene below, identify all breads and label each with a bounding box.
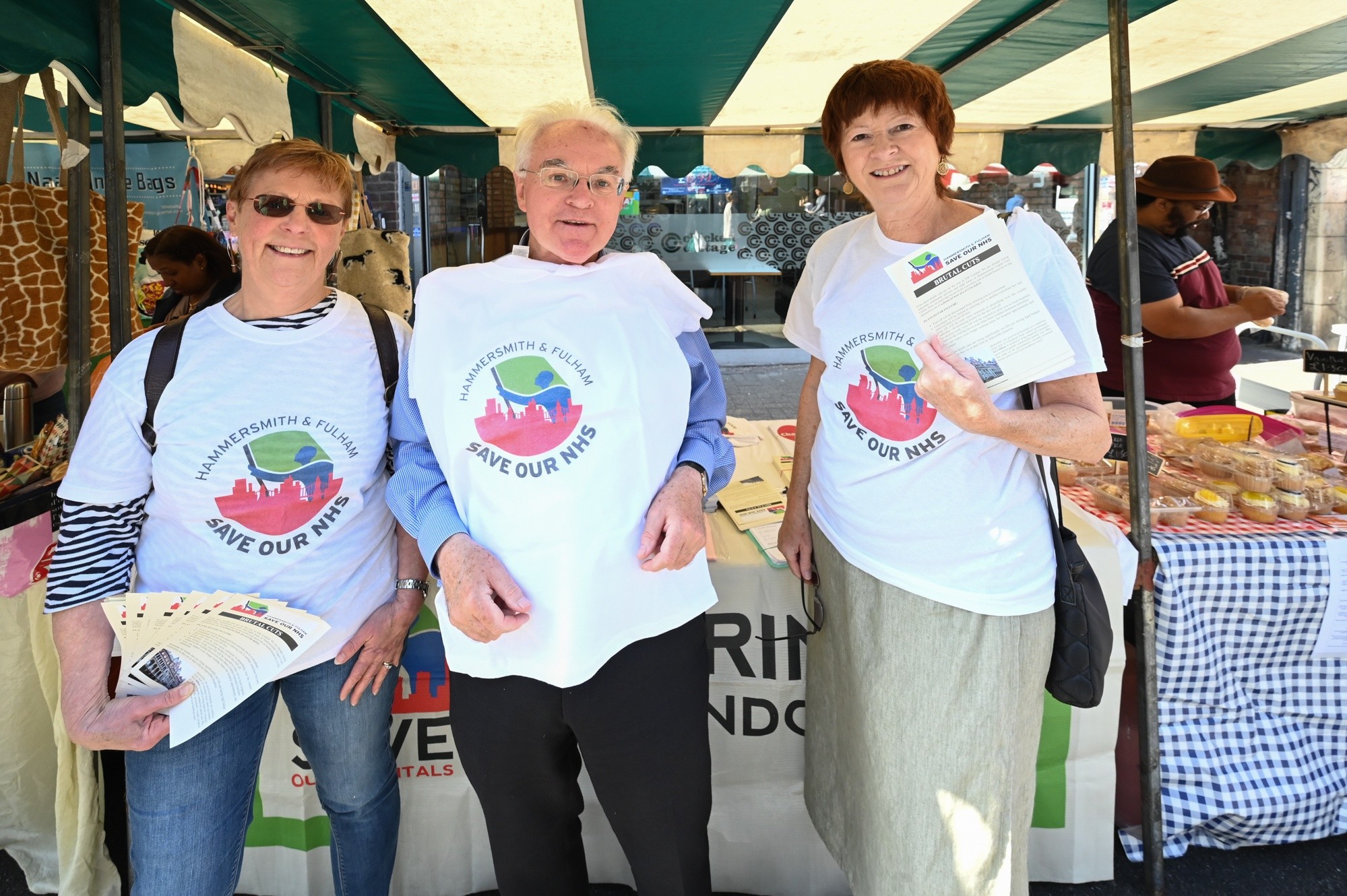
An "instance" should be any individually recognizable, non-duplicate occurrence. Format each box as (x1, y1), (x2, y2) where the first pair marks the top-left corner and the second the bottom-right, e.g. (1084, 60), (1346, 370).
(1054, 438), (1347, 525)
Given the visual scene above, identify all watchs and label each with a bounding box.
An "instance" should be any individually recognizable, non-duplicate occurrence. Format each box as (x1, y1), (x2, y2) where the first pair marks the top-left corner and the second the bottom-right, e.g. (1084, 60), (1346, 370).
(675, 461), (708, 500)
(395, 578), (430, 599)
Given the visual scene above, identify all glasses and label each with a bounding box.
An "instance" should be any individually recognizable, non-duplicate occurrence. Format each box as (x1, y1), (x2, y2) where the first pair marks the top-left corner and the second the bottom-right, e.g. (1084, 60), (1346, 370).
(521, 167), (629, 197)
(1185, 200), (1213, 215)
(755, 561), (825, 641)
(236, 194), (347, 225)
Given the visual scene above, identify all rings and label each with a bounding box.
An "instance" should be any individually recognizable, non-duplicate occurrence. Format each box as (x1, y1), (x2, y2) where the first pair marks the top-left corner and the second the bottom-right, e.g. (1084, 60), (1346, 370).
(382, 661), (393, 669)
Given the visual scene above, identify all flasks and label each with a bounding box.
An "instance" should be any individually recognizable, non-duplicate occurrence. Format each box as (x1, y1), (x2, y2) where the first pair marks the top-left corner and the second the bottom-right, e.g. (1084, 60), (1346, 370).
(3, 383), (32, 451)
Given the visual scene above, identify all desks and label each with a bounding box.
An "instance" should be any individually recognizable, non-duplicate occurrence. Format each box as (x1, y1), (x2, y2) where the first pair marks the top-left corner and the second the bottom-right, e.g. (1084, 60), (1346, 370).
(1055, 436), (1347, 855)
(700, 260), (781, 349)
(237, 418), (1140, 896)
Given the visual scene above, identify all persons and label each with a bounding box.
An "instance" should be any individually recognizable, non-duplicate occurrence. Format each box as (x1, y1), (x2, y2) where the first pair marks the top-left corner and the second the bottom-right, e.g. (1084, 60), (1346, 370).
(779, 60), (1112, 896)
(40, 139), (432, 896)
(387, 99), (737, 896)
(1085, 155), (1289, 410)
(146, 223), (242, 325)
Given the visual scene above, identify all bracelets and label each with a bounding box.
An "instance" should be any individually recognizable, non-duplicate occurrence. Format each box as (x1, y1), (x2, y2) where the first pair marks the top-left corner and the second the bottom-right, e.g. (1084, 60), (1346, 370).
(1235, 285), (1250, 303)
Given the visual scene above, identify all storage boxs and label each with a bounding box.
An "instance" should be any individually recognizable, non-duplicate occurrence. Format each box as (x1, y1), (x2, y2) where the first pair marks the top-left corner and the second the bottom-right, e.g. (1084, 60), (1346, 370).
(1229, 359), (1347, 415)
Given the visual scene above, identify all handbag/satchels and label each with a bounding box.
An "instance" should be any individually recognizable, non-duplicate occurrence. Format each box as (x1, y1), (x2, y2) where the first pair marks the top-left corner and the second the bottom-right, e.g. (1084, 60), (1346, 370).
(1045, 522), (1114, 709)
(134, 274), (200, 316)
(30, 414), (69, 472)
(336, 170), (414, 323)
(0, 68), (144, 375)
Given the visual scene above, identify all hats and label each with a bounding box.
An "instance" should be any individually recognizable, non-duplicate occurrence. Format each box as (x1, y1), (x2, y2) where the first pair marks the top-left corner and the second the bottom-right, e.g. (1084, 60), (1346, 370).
(1136, 155), (1237, 203)
